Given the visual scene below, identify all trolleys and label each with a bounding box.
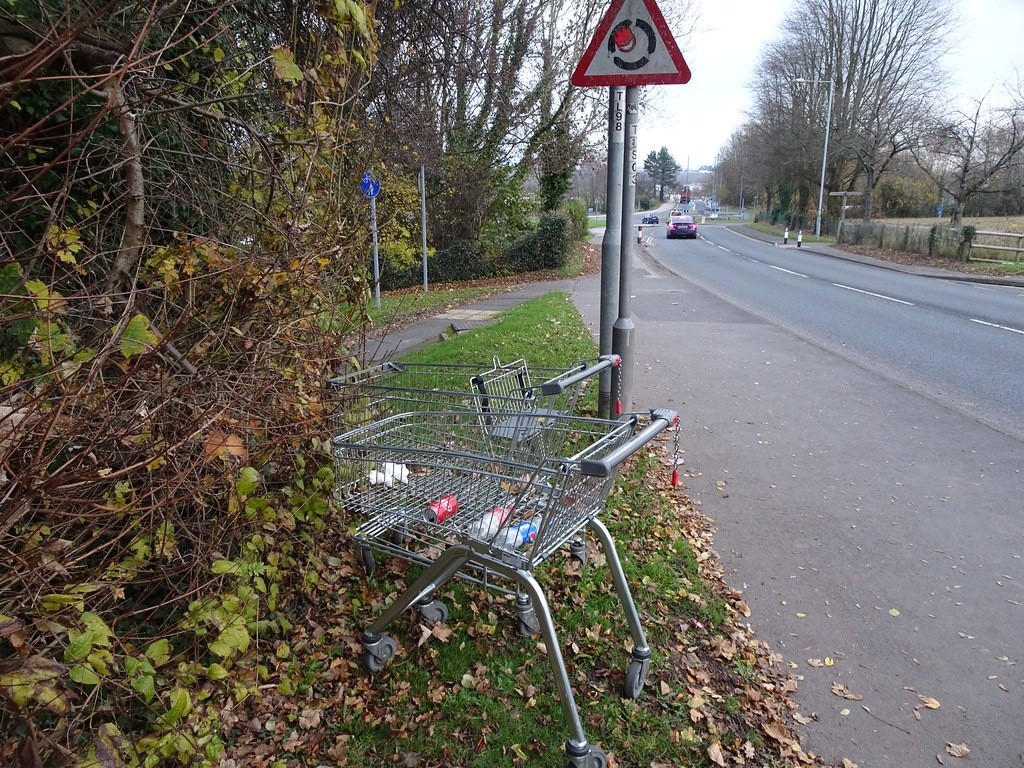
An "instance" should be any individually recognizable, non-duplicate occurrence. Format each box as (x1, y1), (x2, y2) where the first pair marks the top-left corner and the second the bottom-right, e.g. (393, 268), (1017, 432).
(327, 351), (621, 635)
(331, 408), (682, 768)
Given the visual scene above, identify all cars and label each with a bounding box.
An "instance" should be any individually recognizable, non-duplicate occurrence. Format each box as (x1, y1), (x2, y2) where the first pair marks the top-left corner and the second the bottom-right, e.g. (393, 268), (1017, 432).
(706, 201), (720, 211)
(669, 211), (682, 217)
(667, 216), (697, 239)
(642, 213), (660, 224)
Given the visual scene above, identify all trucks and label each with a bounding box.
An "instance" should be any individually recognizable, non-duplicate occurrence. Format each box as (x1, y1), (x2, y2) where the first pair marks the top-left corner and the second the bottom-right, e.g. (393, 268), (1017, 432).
(680, 186), (691, 203)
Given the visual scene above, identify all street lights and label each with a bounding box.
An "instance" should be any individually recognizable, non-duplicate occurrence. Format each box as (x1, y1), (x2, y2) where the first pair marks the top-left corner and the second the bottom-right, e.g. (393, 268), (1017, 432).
(792, 76), (835, 240)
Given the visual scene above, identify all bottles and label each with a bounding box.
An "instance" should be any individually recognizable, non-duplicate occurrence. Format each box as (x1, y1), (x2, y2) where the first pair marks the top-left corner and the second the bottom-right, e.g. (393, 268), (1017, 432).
(498, 517), (554, 549)
(468, 506), (517, 541)
